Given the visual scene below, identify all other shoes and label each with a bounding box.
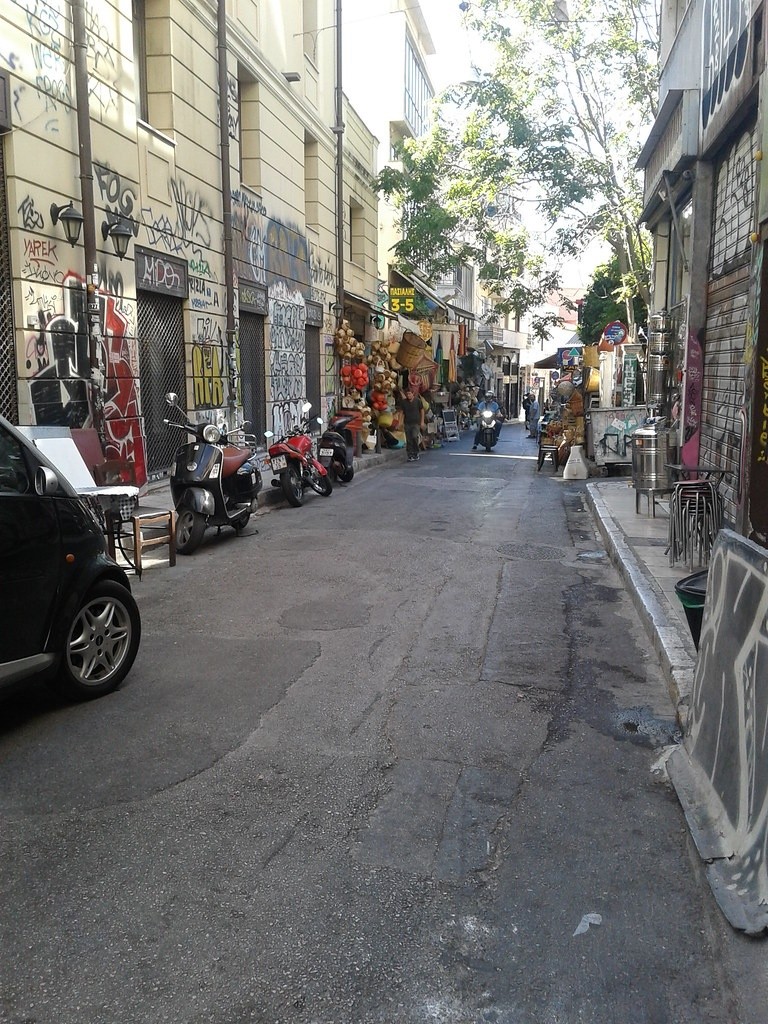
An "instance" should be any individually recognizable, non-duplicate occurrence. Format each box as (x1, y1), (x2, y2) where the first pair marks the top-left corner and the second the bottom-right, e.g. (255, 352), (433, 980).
(526, 434), (538, 439)
(472, 446), (477, 449)
(407, 455), (420, 462)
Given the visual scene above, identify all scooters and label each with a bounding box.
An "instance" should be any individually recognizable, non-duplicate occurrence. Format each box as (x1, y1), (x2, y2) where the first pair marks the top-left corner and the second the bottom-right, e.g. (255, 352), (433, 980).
(162, 392), (263, 554)
(319, 411), (369, 482)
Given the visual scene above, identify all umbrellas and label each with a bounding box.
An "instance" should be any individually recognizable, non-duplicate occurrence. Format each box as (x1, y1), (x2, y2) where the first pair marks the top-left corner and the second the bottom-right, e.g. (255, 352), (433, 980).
(448, 334), (457, 408)
(436, 334), (444, 384)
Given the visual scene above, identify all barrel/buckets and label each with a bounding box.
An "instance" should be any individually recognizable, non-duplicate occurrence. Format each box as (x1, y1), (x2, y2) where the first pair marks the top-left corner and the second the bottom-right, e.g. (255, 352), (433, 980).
(632, 423), (677, 490)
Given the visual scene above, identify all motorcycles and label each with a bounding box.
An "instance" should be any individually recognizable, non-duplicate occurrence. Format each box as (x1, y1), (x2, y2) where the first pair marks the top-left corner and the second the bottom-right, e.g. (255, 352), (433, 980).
(471, 405), (504, 452)
(264, 402), (333, 507)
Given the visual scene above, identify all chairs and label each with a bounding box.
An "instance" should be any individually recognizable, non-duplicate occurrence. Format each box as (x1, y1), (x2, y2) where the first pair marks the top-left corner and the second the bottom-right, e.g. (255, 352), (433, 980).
(92, 459), (176, 576)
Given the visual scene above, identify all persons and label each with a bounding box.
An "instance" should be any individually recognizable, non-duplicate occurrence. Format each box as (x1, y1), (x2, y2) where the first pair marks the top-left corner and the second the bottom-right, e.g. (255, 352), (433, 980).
(523, 393), (540, 438)
(398, 389), (424, 462)
(472, 390), (502, 449)
(366, 385), (399, 448)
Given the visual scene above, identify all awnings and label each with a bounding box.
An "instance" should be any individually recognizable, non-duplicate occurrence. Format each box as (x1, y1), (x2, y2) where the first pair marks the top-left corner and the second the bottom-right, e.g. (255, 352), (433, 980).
(393, 269), (455, 325)
(534, 353), (557, 369)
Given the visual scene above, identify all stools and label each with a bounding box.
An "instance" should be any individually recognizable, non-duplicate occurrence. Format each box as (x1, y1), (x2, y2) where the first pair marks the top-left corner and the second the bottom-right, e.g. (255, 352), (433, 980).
(664, 479), (726, 573)
(537, 444), (560, 474)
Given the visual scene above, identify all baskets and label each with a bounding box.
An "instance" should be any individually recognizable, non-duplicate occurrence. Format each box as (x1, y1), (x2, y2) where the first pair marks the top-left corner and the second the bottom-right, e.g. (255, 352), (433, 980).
(566, 391), (584, 417)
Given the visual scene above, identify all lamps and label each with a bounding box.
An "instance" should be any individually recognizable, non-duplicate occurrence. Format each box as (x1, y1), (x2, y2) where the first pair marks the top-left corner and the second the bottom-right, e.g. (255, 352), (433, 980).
(50, 200), (85, 249)
(101, 219), (133, 261)
(343, 304), (356, 323)
(329, 300), (344, 321)
(370, 313), (382, 330)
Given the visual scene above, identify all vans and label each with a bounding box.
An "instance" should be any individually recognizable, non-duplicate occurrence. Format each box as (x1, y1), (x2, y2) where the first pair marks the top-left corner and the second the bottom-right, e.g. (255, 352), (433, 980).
(0, 416), (141, 703)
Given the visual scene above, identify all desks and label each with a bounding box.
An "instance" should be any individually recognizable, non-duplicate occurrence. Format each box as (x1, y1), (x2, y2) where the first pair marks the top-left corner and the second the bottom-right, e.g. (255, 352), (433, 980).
(663, 463), (735, 490)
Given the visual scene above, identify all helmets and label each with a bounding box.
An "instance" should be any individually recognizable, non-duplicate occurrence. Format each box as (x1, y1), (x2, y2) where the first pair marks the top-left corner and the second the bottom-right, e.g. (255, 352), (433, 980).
(488, 390), (493, 394)
(485, 393), (492, 398)
